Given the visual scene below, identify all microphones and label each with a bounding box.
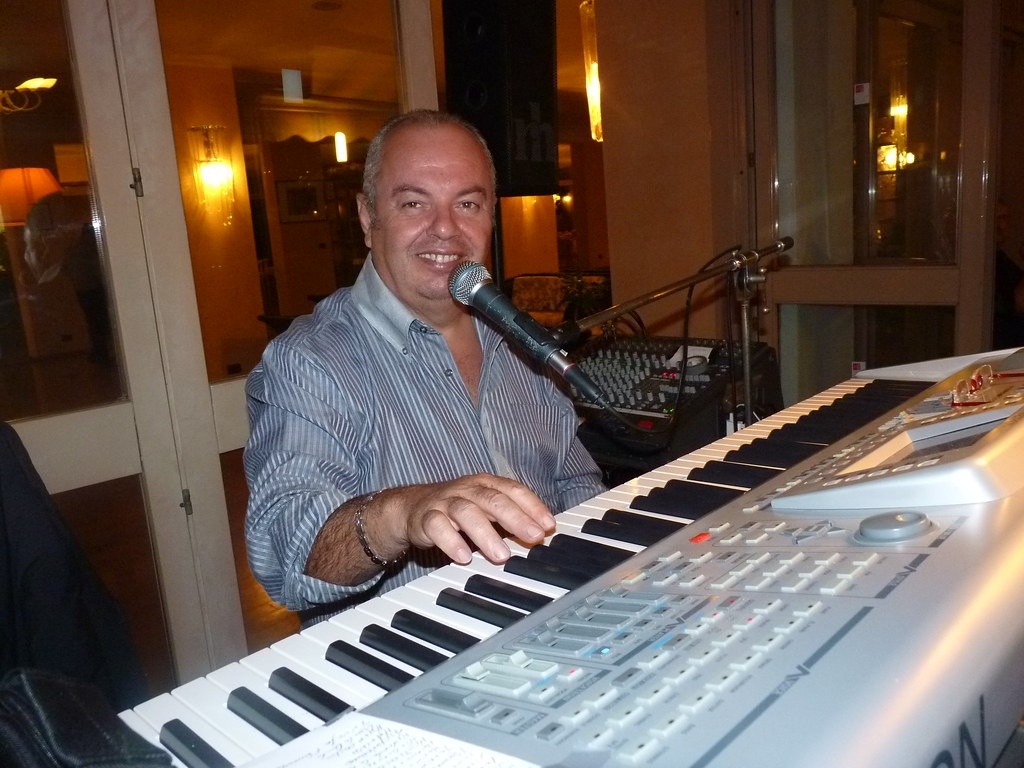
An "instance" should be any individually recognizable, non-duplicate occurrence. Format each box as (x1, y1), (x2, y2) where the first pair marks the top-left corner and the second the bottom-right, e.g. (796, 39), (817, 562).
(448, 260), (610, 410)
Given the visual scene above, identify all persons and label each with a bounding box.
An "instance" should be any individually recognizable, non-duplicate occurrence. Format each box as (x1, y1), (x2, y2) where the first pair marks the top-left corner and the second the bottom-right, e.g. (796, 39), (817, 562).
(0, 422), (150, 714)
(240, 110), (608, 634)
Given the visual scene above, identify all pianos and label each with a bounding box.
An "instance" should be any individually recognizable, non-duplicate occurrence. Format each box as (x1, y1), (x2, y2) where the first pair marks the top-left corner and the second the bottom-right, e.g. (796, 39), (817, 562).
(114, 346), (1024, 768)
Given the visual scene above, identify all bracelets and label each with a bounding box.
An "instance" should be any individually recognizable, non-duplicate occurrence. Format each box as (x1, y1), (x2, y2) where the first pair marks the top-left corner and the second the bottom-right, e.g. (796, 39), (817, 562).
(354, 486), (408, 566)
